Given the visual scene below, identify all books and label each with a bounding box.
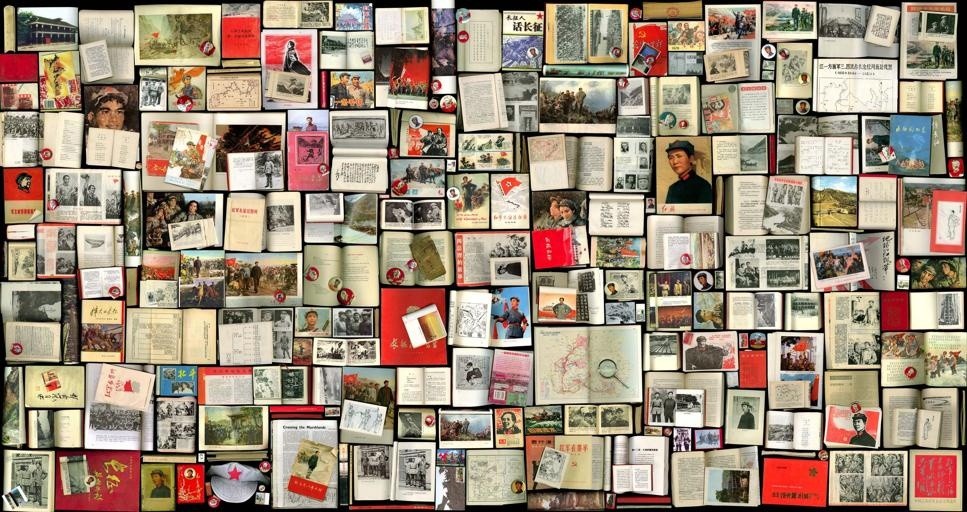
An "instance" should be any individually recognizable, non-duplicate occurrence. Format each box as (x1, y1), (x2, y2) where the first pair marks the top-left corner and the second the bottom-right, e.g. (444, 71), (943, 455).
(0, 2), (967, 512)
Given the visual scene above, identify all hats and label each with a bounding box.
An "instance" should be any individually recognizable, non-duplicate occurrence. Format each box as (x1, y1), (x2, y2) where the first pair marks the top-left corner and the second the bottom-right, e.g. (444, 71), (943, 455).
(15, 173), (33, 184)
(182, 74), (192, 82)
(924, 259), (957, 275)
(510, 296), (520, 302)
(852, 413), (868, 423)
(697, 273), (706, 278)
(742, 401), (750, 405)
(667, 140), (695, 157)
(150, 469), (164, 477)
(206, 462), (270, 504)
(696, 336), (708, 342)
(550, 195), (576, 211)
(339, 72), (362, 80)
(155, 195), (177, 211)
(86, 85), (130, 109)
(305, 309), (318, 319)
